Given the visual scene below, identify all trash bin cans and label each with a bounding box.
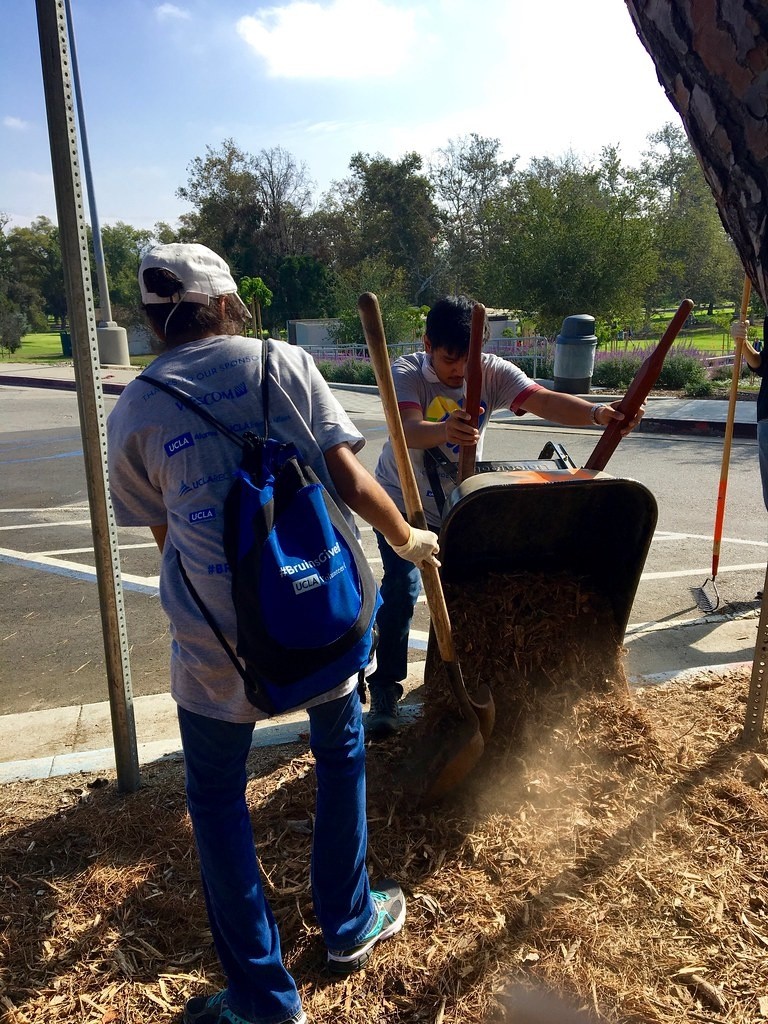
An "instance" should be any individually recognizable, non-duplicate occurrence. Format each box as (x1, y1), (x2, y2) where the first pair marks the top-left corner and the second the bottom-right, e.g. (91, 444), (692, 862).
(552, 312), (599, 395)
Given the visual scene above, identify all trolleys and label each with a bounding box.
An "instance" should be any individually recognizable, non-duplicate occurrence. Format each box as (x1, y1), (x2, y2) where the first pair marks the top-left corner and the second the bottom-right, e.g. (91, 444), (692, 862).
(424, 297), (695, 687)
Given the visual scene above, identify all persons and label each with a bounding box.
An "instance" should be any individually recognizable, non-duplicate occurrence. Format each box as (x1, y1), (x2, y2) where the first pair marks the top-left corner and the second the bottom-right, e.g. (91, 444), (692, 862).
(106, 243), (445, 1024)
(364, 296), (647, 732)
(753, 338), (762, 351)
(731, 316), (768, 598)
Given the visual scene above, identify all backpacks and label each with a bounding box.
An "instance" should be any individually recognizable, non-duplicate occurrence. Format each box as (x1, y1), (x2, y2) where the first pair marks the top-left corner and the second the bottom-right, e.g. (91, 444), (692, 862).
(133, 341), (382, 714)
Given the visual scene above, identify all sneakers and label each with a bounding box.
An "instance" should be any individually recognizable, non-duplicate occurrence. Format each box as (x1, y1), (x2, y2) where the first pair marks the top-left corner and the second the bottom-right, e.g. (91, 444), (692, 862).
(365, 683), (404, 731)
(183, 988), (307, 1024)
(328, 879), (406, 974)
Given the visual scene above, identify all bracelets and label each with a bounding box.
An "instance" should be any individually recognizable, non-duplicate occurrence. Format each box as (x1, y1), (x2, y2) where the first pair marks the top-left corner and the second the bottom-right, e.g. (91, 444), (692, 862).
(590, 403), (605, 427)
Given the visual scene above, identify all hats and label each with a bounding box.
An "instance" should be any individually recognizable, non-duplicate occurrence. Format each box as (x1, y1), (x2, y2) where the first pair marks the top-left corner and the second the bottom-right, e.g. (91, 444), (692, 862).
(138, 243), (254, 320)
